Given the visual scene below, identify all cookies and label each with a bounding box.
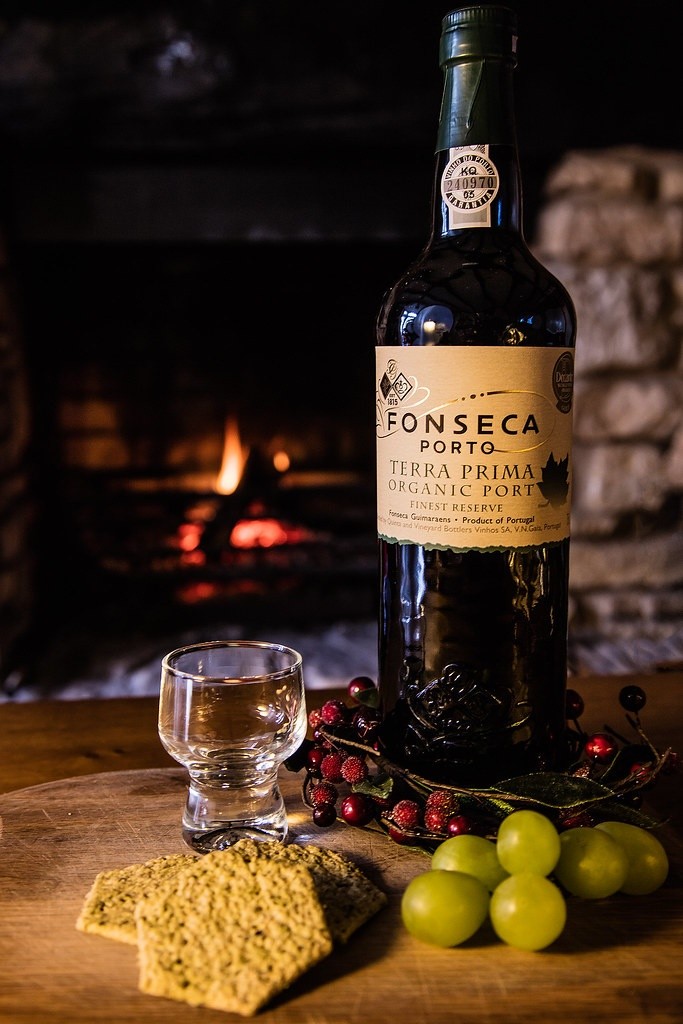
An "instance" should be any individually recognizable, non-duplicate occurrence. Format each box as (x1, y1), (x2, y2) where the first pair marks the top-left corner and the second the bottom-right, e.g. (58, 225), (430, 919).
(77, 838), (388, 1017)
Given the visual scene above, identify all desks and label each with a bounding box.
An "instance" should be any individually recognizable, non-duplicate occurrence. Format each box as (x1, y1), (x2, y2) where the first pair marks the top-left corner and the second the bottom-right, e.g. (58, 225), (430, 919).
(0, 671), (683, 1024)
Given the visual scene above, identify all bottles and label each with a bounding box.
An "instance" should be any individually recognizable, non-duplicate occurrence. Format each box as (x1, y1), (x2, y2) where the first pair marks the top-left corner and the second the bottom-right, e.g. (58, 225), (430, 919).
(375, 7), (576, 788)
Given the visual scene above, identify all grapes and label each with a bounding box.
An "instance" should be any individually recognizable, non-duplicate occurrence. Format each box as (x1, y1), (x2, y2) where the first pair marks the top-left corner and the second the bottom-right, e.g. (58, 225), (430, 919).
(400, 807), (669, 951)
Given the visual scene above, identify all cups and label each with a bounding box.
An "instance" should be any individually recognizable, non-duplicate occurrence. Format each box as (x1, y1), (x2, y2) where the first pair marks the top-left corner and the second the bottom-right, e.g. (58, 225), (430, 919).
(158, 640), (308, 854)
